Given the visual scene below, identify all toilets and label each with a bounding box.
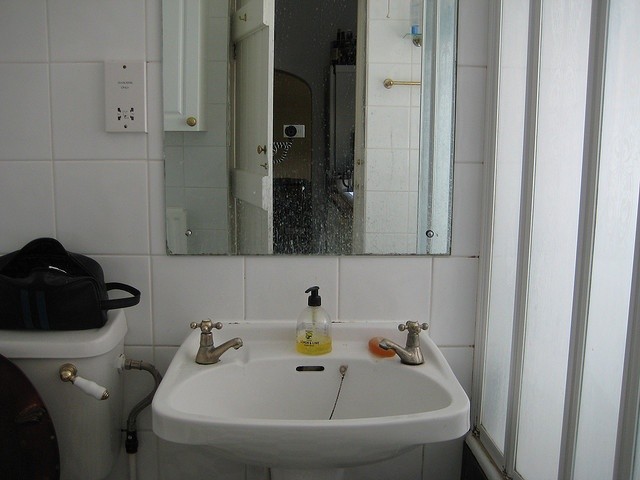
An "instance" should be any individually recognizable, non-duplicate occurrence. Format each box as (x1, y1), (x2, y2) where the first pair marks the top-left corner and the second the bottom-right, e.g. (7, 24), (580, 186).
(0, 308), (127, 480)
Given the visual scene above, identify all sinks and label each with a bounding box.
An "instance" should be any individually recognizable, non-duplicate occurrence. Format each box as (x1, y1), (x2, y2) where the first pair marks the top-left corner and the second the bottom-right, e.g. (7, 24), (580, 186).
(151, 318), (471, 479)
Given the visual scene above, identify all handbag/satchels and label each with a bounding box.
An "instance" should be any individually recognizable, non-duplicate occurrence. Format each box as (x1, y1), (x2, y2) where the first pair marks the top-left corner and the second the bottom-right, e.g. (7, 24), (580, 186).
(0, 237), (140, 331)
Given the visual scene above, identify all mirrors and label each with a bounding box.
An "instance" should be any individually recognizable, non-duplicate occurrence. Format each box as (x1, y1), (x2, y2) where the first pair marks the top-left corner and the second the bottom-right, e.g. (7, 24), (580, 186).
(160, 1), (459, 255)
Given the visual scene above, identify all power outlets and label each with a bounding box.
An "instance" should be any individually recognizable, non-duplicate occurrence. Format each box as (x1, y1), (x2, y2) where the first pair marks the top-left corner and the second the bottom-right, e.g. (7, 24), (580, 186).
(103, 56), (148, 135)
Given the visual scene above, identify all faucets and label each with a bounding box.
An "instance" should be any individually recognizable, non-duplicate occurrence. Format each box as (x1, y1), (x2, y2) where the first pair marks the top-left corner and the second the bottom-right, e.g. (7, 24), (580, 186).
(377, 320), (429, 366)
(189, 318), (244, 364)
